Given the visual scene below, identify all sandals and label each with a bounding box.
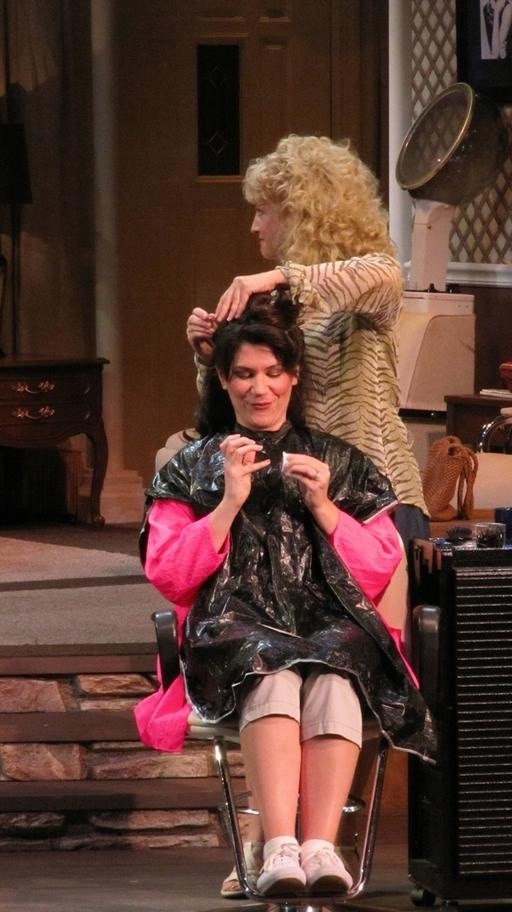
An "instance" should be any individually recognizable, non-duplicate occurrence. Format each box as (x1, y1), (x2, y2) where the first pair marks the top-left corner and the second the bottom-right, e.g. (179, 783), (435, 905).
(220, 840), (262, 897)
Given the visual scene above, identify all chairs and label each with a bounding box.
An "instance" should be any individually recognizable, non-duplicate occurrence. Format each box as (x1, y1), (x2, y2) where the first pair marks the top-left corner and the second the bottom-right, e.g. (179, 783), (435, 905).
(152, 605), (439, 912)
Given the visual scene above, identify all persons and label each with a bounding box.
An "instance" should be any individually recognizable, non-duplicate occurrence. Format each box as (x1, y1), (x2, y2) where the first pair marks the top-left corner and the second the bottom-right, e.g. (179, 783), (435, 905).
(139, 292), (428, 896)
(182, 132), (424, 900)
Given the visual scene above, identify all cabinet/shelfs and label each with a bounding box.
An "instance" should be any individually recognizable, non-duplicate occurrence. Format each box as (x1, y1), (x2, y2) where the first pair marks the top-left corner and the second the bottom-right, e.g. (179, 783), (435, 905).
(408, 538), (512, 912)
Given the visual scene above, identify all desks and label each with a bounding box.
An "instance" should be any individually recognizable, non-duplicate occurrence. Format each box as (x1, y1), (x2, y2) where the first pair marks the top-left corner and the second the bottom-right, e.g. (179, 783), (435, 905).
(1, 358), (110, 531)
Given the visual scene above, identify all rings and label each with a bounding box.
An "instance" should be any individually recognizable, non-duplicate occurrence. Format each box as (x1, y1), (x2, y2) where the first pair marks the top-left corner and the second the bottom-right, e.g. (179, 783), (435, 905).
(315, 471), (320, 481)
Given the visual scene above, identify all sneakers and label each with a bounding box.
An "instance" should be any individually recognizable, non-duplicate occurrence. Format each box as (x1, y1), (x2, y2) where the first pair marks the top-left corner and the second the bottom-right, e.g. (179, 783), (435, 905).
(304, 850), (353, 896)
(257, 841), (306, 895)
